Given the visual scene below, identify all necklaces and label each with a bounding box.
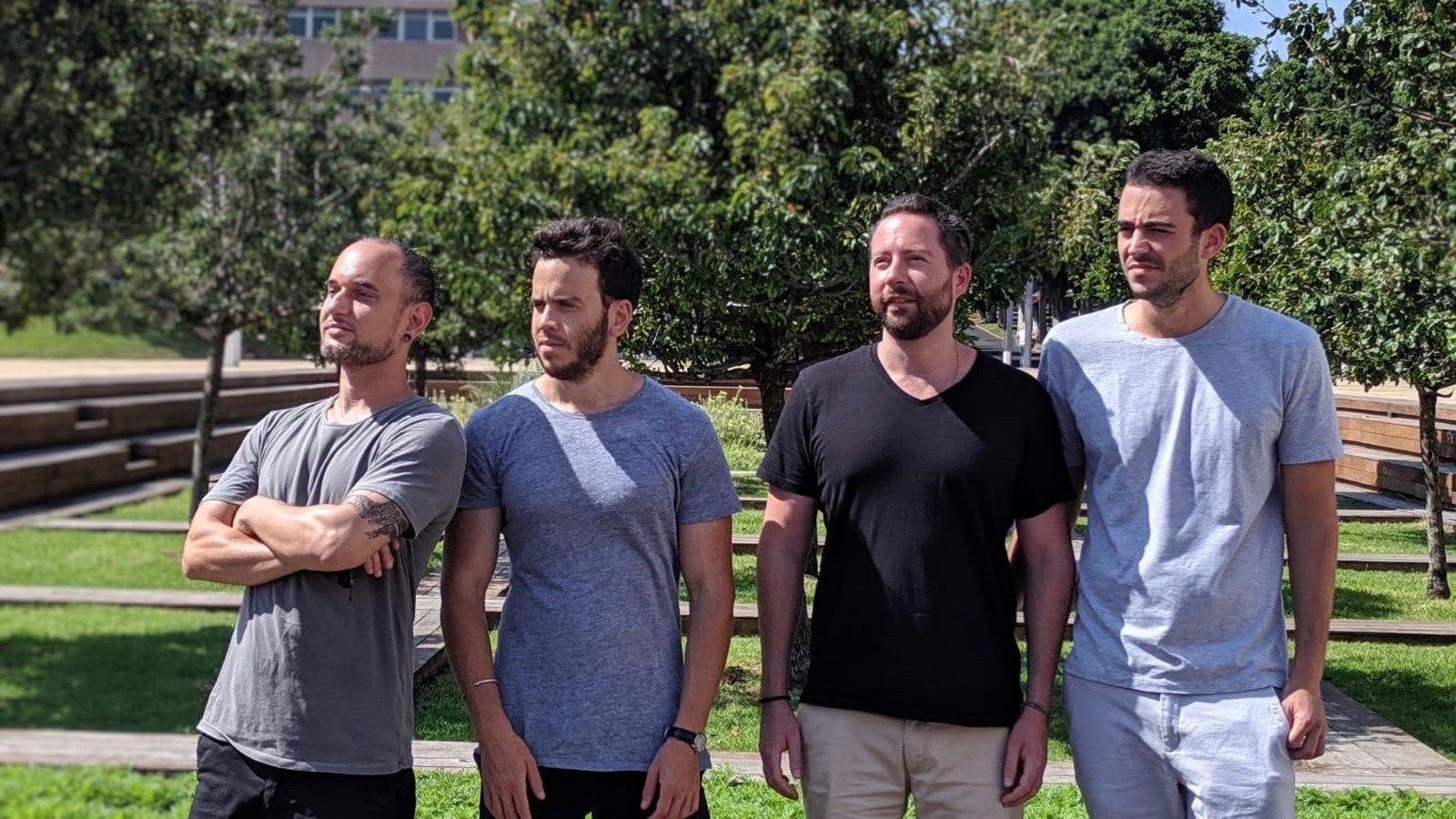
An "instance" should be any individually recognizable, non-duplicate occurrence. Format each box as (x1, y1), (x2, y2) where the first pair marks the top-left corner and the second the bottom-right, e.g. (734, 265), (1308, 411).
(948, 339), (960, 389)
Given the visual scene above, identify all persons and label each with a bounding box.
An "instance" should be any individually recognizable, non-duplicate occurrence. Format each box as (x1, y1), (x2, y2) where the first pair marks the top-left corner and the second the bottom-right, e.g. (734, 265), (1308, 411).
(1040, 149), (1341, 819)
(757, 195), (1071, 819)
(183, 236), (468, 819)
(440, 218), (740, 818)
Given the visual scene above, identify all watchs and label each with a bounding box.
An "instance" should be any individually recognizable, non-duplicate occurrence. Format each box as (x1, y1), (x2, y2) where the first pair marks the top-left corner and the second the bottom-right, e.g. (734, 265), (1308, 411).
(664, 725), (708, 755)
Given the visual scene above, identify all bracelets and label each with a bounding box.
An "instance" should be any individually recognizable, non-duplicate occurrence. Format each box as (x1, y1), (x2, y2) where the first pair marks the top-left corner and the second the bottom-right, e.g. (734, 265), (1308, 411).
(756, 694), (792, 707)
(1024, 699), (1052, 718)
(459, 678), (500, 702)
